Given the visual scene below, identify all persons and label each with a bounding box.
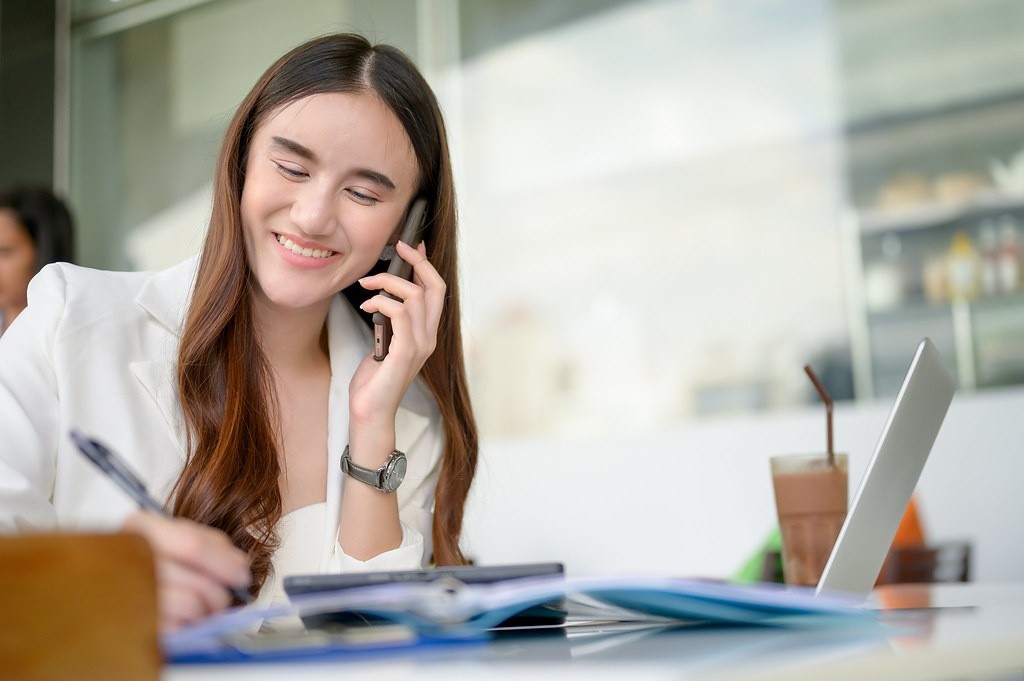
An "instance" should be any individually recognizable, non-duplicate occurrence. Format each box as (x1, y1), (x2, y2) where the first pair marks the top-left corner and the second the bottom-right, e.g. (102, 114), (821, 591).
(0, 33), (479, 644)
(0, 181), (76, 337)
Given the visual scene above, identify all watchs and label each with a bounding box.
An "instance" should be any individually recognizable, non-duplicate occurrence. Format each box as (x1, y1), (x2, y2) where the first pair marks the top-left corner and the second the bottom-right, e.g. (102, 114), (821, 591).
(340, 445), (410, 495)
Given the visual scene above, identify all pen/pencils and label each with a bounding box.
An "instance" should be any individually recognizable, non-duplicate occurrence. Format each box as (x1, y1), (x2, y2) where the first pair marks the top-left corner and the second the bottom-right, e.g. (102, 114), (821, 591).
(71, 432), (255, 605)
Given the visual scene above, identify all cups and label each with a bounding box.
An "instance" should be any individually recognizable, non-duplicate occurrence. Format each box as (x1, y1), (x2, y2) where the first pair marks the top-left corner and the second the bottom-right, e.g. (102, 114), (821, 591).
(770, 451), (850, 587)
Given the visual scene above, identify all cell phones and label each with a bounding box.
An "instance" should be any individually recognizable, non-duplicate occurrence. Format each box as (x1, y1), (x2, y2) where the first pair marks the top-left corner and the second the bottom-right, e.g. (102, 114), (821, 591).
(374, 200), (430, 360)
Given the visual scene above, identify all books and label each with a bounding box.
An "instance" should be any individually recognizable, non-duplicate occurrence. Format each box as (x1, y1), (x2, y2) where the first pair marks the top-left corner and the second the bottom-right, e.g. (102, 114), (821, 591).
(280, 562), (571, 639)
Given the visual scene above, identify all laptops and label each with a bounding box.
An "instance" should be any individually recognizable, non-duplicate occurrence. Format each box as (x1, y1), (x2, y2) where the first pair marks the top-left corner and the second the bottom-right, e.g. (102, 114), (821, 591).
(812, 341), (957, 593)
